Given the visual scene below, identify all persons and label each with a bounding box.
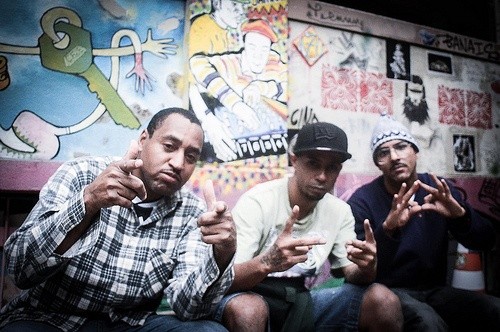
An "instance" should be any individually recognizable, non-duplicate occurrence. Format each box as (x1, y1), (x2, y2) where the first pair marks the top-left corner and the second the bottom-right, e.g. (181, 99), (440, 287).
(347, 112), (500, 332)
(0, 107), (238, 332)
(214, 123), (404, 332)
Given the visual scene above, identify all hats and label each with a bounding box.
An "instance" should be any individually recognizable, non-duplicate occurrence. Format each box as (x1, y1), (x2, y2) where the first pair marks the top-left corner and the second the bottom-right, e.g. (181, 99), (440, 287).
(292, 122), (352, 163)
(370, 113), (419, 160)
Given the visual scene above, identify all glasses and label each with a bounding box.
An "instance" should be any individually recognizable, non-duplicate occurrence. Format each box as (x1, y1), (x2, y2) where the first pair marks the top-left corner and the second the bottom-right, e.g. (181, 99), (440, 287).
(374, 142), (411, 165)
